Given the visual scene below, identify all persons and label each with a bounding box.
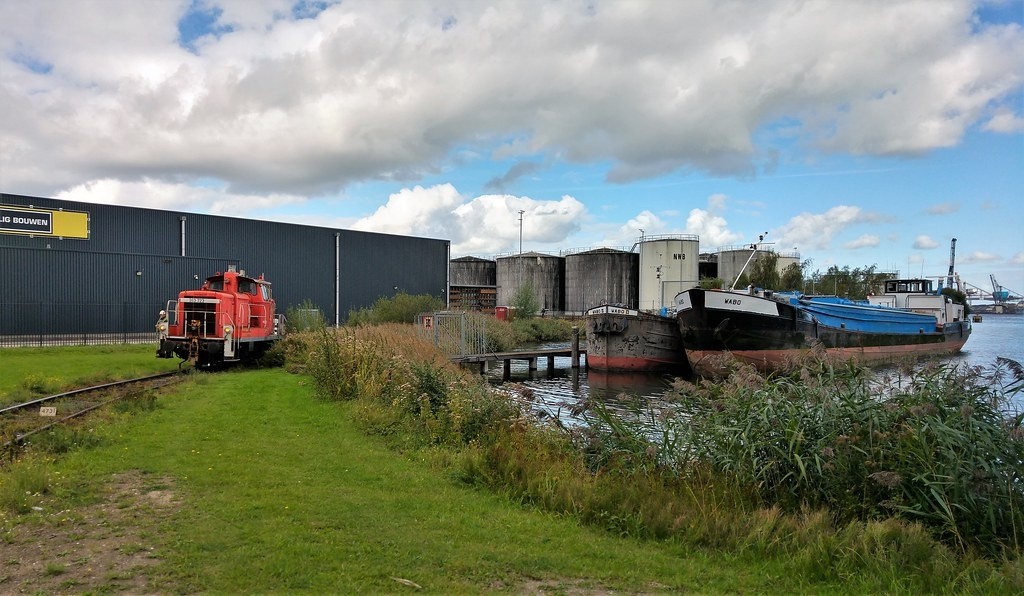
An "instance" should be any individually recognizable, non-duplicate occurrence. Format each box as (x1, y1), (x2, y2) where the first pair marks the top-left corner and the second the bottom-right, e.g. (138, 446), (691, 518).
(156, 310), (172, 358)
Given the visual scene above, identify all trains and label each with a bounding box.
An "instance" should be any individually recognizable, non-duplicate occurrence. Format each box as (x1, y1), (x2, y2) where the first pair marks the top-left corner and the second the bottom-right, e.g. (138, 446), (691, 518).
(165, 269), (290, 375)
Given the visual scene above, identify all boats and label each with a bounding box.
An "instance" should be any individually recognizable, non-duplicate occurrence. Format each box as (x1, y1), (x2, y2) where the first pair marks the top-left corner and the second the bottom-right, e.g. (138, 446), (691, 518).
(585, 298), (680, 374)
(953, 272), (1024, 315)
(674, 232), (975, 385)
